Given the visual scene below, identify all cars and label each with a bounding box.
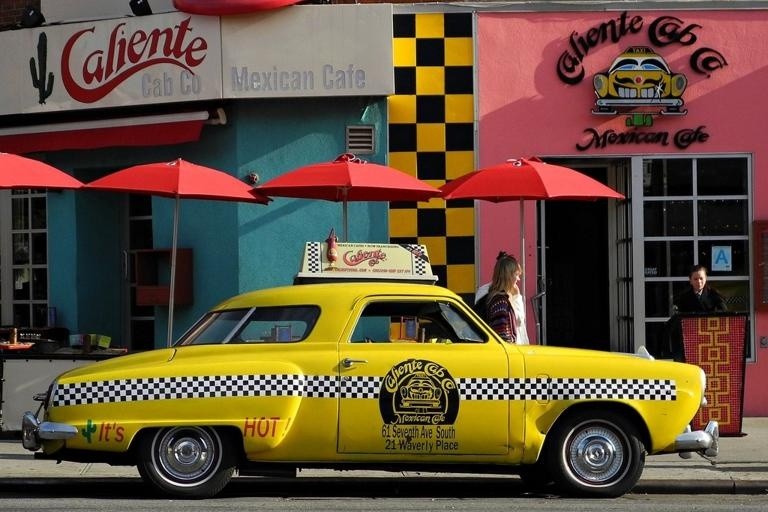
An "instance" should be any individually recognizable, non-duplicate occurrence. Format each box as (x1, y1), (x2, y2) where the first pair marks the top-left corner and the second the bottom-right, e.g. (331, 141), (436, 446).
(17, 239), (721, 501)
(703, 270), (749, 315)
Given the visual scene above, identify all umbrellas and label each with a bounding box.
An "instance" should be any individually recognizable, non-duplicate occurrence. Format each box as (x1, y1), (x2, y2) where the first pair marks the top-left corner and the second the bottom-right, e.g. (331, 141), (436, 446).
(436, 156), (626, 325)
(0, 149), (88, 191)
(248, 151), (444, 245)
(83, 159), (272, 347)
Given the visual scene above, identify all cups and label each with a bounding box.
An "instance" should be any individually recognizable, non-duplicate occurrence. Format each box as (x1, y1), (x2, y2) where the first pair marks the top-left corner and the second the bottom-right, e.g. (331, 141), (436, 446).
(9, 327), (19, 344)
(416, 327), (426, 342)
(46, 306), (56, 328)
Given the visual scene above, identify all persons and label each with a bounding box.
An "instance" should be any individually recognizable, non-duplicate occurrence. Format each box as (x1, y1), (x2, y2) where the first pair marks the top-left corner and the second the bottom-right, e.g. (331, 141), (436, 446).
(475, 255), (522, 345)
(673, 264), (729, 316)
(475, 249), (531, 346)
